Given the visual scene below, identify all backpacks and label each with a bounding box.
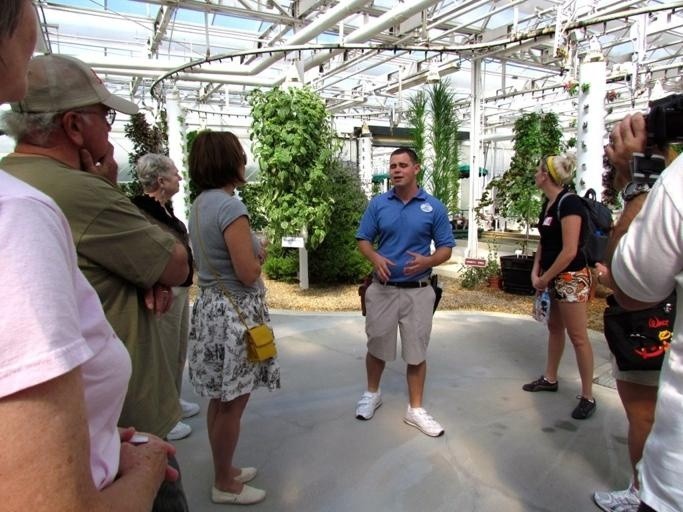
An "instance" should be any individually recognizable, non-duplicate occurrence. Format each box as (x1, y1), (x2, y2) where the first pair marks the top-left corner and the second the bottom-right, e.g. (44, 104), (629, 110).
(557, 188), (614, 269)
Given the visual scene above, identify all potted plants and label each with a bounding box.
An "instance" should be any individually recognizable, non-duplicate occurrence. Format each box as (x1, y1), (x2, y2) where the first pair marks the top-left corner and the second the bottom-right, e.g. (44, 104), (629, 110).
(485, 227), (504, 290)
(472, 107), (578, 296)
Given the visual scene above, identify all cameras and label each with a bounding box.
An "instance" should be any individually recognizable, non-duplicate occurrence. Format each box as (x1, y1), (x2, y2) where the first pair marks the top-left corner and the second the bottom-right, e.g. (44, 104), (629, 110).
(643, 94), (683, 147)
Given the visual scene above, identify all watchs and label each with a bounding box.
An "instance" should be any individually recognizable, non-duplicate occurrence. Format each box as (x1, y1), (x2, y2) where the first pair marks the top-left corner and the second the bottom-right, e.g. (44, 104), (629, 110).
(621, 180), (652, 202)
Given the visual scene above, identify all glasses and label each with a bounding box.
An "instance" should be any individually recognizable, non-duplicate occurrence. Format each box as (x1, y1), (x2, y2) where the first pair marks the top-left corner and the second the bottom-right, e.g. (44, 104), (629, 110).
(76, 108), (116, 123)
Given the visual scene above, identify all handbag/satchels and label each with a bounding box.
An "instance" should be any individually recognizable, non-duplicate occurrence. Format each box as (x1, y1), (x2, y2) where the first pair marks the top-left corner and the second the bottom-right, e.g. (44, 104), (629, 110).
(603, 291), (677, 371)
(245, 325), (278, 364)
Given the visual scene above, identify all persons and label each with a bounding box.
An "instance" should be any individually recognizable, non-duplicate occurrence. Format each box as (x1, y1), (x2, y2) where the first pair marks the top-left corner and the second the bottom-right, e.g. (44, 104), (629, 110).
(0, 0), (180, 512)
(186, 130), (281, 505)
(603, 112), (683, 512)
(591, 262), (677, 512)
(522, 153), (597, 419)
(127, 154), (201, 440)
(354, 148), (455, 437)
(0, 51), (193, 512)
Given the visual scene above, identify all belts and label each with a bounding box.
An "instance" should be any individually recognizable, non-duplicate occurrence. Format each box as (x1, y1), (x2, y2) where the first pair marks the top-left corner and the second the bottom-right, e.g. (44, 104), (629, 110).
(378, 279), (428, 289)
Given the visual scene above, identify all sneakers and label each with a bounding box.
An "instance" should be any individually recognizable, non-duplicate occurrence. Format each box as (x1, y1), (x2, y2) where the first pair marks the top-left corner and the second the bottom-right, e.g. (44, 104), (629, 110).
(593, 485), (639, 511)
(167, 422), (192, 441)
(212, 484), (266, 505)
(179, 399), (200, 418)
(403, 402), (444, 437)
(233, 467), (257, 483)
(356, 389), (383, 419)
(523, 377), (559, 392)
(571, 395), (596, 419)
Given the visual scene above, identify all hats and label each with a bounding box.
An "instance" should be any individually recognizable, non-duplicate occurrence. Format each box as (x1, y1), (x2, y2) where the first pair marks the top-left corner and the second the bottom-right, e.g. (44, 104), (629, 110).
(546, 155), (574, 185)
(10, 50), (139, 118)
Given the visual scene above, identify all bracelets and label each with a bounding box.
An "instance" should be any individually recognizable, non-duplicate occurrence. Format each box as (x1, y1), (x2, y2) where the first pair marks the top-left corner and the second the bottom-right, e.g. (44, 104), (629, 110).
(597, 272), (602, 285)
